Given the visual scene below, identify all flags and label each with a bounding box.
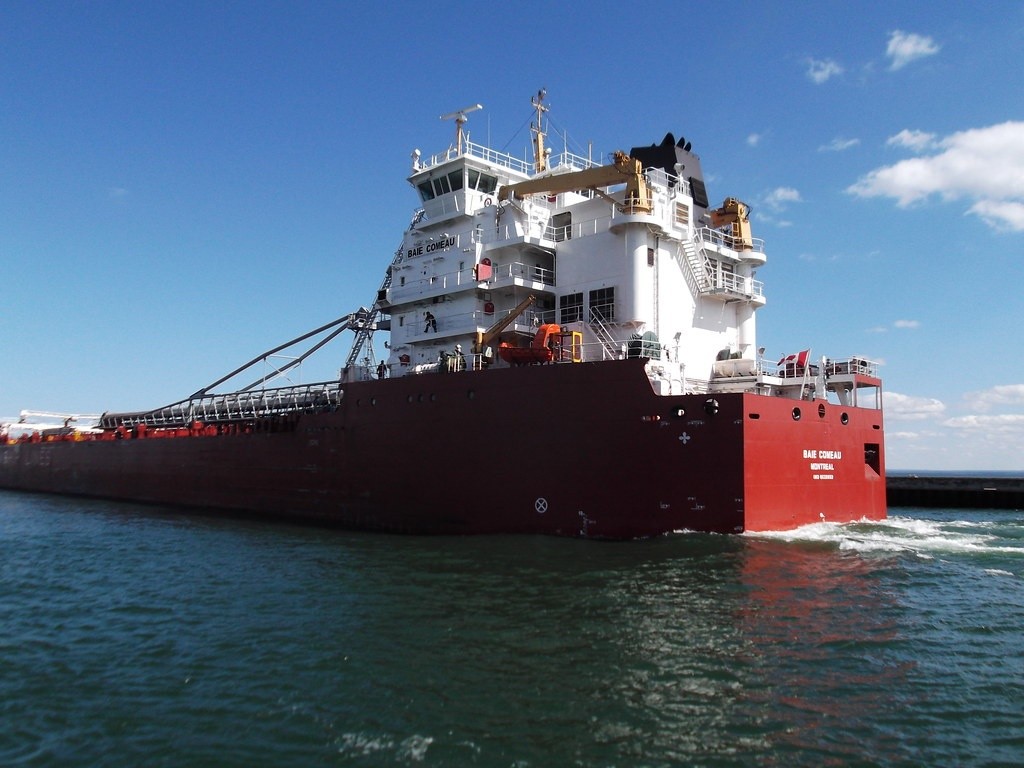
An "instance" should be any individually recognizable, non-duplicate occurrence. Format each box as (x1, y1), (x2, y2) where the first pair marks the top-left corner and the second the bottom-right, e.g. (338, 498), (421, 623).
(777, 349), (809, 367)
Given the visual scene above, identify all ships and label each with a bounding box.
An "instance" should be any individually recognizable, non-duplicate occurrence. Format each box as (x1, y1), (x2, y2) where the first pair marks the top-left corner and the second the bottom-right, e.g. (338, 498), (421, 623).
(0, 87), (888, 545)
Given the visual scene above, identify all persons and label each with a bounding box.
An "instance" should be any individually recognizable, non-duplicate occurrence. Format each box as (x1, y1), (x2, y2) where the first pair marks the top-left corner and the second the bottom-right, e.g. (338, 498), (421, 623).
(455, 344), (467, 371)
(424, 311), (437, 333)
(439, 350), (449, 373)
(377, 360), (387, 381)
